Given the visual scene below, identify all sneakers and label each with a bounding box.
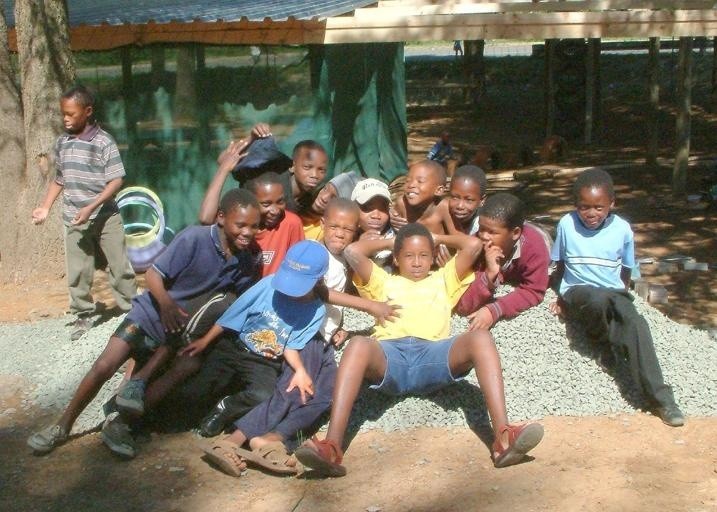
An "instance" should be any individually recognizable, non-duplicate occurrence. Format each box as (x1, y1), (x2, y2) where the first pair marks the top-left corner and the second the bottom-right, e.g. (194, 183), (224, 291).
(116, 381), (145, 417)
(70, 319), (91, 341)
(100, 417), (135, 458)
(656, 403), (684, 426)
(28, 424), (67, 453)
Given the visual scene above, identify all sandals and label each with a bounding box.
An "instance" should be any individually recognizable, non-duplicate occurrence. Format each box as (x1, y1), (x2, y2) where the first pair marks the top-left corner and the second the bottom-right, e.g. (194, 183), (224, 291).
(492, 422), (544, 467)
(296, 436), (345, 477)
(238, 443), (296, 474)
(204, 433), (242, 477)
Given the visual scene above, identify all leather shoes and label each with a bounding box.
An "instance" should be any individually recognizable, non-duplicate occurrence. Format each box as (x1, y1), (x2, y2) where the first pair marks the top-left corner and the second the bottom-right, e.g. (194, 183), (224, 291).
(200, 396), (228, 437)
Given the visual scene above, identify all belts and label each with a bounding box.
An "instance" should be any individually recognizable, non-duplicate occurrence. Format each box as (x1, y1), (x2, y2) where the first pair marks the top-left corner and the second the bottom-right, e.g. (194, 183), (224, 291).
(235, 337), (254, 355)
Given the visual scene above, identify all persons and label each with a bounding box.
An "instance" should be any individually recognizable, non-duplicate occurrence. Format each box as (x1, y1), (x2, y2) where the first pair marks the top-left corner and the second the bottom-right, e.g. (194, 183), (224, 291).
(31, 85), (140, 330)
(26, 123), (555, 481)
(548, 168), (687, 427)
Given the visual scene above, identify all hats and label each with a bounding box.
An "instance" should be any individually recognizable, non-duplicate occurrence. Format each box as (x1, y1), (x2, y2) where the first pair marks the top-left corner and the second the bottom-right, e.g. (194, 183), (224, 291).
(271, 240), (329, 298)
(329, 171), (359, 198)
(351, 179), (392, 205)
(232, 137), (293, 181)
